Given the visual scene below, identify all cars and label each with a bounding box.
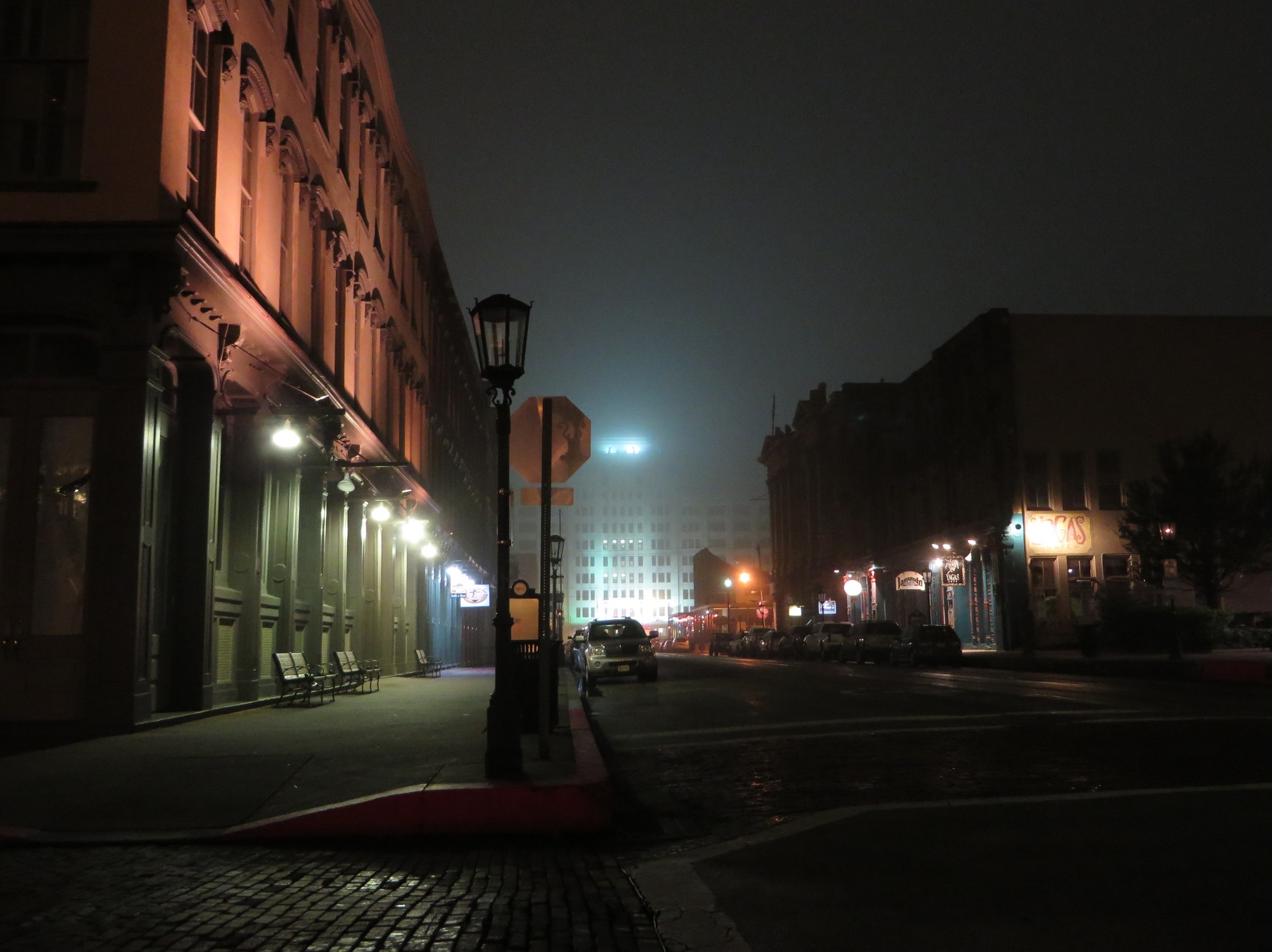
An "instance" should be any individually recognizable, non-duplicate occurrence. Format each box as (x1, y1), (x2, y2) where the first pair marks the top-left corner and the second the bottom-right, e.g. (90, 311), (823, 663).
(731, 627), (814, 662)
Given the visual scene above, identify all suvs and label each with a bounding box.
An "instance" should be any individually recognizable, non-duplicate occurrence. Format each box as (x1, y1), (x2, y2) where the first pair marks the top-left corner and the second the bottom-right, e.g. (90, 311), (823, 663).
(837, 619), (901, 664)
(802, 620), (855, 661)
(652, 637), (691, 652)
(890, 622), (963, 668)
(567, 616), (660, 685)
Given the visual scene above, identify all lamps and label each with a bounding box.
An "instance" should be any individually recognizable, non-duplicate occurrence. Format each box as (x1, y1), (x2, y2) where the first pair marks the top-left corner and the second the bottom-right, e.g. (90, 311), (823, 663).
(272, 418), (300, 450)
(1010, 522), (1021, 529)
(964, 546), (974, 562)
(337, 472), (356, 492)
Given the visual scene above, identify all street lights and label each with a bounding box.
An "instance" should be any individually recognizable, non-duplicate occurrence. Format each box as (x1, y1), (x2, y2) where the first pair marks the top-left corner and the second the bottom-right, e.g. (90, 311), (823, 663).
(460, 286), (536, 778)
(818, 589), (825, 622)
(844, 579), (862, 619)
(758, 602), (765, 627)
(548, 531), (565, 671)
(965, 537), (992, 647)
(724, 578), (732, 637)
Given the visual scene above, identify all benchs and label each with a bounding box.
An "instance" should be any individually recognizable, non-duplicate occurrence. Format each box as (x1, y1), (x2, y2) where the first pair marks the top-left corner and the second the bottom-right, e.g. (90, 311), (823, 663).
(270, 650), (383, 709)
(414, 649), (443, 680)
